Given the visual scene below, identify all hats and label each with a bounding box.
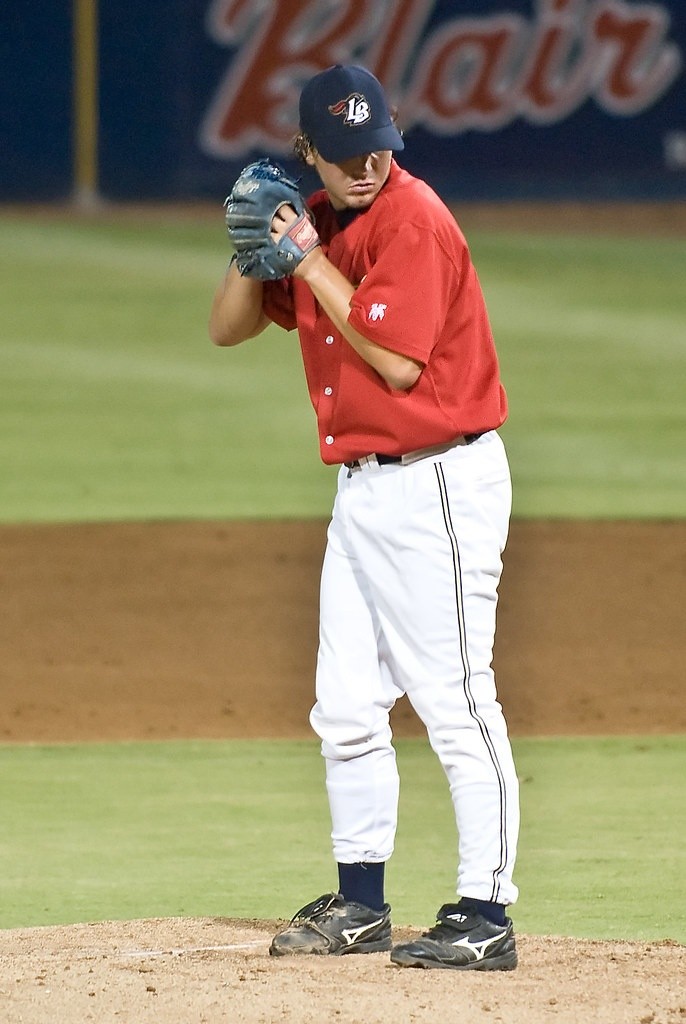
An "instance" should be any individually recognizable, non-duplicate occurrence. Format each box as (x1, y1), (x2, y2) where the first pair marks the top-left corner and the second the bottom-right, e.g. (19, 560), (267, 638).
(299, 63), (405, 164)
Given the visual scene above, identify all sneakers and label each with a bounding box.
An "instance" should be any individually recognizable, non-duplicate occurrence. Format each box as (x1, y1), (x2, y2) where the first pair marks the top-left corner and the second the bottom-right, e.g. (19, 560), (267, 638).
(390, 902), (518, 971)
(268, 892), (393, 957)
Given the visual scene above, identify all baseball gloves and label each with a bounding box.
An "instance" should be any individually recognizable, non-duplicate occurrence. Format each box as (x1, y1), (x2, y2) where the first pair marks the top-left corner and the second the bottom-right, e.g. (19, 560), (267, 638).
(224, 155), (323, 283)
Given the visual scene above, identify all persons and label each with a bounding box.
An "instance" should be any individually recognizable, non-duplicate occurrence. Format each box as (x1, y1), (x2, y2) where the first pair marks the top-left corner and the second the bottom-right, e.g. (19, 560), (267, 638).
(208, 64), (521, 970)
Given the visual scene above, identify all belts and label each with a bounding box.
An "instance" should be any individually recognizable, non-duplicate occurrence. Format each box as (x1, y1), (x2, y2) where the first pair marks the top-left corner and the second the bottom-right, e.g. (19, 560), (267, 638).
(353, 433), (487, 467)
